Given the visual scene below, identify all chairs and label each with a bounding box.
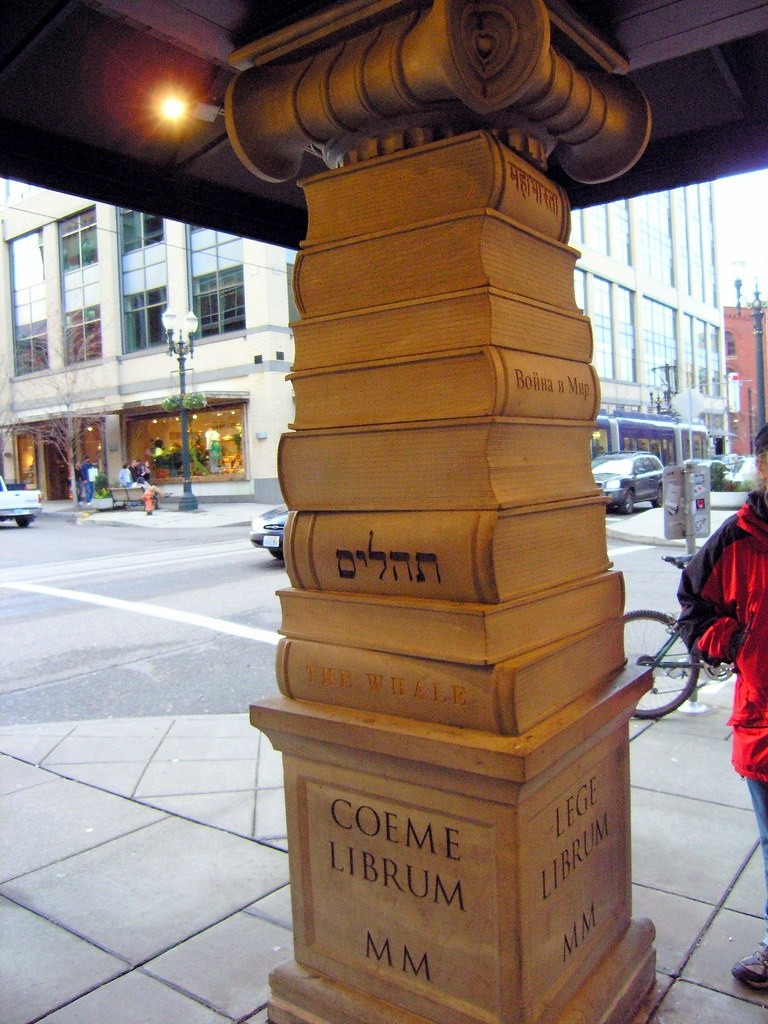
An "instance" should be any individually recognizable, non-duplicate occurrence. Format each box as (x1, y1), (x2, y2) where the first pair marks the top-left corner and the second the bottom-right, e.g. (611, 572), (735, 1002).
(145, 448), (209, 478)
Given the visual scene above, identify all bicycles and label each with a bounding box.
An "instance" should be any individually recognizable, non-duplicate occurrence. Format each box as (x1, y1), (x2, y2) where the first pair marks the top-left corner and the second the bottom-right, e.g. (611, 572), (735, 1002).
(624, 554), (738, 720)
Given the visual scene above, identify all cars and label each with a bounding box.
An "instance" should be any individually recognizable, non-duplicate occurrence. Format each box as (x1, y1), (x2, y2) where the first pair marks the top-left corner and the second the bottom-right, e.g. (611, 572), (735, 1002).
(686, 453), (757, 489)
(247, 506), (287, 561)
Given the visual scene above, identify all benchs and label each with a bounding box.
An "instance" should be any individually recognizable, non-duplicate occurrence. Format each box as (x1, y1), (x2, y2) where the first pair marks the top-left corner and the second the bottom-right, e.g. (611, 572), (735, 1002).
(106, 487), (159, 512)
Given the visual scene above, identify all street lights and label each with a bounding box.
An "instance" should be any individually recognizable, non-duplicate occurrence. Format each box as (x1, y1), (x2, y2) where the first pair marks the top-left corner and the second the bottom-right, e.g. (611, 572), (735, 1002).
(649, 363), (683, 463)
(733, 260), (765, 433)
(159, 307), (201, 509)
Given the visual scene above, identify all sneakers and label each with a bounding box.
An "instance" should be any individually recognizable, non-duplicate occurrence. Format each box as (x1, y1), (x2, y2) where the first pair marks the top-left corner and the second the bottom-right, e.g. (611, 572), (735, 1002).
(731, 943), (768, 989)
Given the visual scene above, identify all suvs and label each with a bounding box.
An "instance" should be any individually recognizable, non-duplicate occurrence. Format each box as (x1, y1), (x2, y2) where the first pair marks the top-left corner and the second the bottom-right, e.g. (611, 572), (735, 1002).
(590, 450), (665, 515)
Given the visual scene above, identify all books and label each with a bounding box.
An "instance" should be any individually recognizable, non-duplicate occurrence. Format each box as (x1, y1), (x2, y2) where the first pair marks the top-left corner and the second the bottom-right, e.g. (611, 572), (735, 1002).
(272, 129), (629, 737)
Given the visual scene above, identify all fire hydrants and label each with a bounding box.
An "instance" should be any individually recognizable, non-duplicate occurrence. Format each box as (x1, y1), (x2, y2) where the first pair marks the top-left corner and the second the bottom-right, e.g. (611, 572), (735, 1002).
(141, 489), (155, 515)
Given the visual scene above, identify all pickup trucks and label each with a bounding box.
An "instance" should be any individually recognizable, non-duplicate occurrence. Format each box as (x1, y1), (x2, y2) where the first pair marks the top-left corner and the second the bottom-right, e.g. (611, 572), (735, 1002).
(0, 475), (43, 527)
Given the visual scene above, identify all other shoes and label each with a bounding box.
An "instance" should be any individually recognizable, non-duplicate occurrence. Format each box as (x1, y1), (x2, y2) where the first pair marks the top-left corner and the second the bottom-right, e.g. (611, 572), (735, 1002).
(164, 493), (172, 498)
(155, 506), (161, 509)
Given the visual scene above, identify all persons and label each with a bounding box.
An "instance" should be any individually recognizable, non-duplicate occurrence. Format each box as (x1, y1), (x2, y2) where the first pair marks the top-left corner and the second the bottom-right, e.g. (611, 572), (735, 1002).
(73, 455), (97, 505)
(205, 428), (223, 475)
(680, 421), (768, 991)
(120, 461), (172, 506)
(154, 436), (164, 473)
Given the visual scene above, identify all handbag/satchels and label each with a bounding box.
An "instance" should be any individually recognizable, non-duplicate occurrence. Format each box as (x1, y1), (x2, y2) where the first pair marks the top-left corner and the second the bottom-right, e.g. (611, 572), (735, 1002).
(88, 465), (98, 482)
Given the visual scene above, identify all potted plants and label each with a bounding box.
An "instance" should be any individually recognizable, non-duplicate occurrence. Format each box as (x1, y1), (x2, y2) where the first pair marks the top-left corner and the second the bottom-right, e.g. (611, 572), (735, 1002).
(92, 471), (113, 511)
